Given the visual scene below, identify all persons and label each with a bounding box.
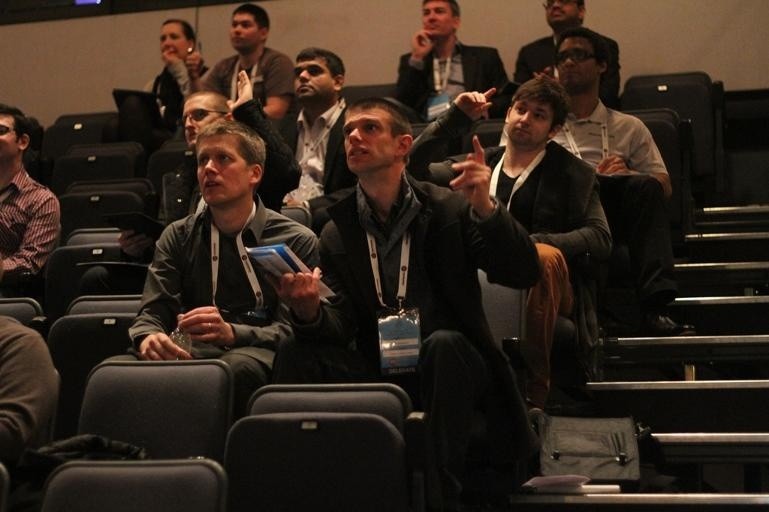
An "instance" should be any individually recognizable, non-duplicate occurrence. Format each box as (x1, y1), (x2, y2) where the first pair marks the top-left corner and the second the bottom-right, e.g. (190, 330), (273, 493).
(0, 102), (61, 298)
(0, 314), (63, 512)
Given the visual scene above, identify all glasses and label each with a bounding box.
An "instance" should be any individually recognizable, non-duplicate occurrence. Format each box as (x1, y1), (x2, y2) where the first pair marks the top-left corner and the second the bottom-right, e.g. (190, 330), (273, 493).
(558, 49), (594, 62)
(175, 109), (228, 126)
(543, 0), (570, 8)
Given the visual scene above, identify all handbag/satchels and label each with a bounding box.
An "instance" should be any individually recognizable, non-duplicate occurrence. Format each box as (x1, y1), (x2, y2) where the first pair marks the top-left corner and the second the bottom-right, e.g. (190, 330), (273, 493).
(528, 407), (652, 483)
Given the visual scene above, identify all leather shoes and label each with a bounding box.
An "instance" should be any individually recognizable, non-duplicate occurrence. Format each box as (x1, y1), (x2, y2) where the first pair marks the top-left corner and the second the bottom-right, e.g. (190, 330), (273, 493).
(645, 310), (702, 335)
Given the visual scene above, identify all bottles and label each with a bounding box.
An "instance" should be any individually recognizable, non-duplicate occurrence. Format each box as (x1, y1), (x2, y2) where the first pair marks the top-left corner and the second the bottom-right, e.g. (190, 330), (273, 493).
(167, 311), (192, 360)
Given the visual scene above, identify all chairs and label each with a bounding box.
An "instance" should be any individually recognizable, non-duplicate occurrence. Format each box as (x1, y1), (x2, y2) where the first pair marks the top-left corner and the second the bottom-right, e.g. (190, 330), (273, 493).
(1, 70), (731, 511)
